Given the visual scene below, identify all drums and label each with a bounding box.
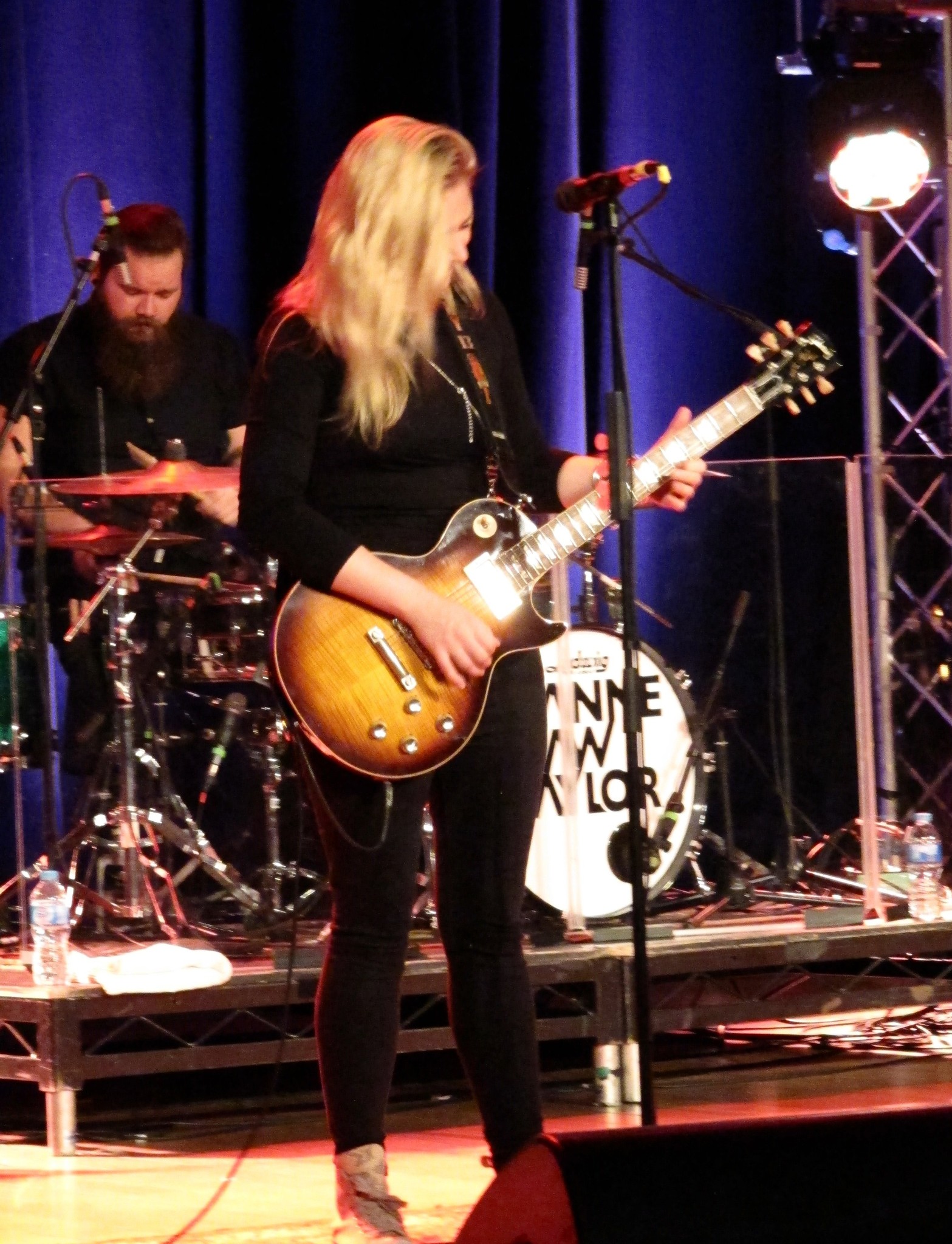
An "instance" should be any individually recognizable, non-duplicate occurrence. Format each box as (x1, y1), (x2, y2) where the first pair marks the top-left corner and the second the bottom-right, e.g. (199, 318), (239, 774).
(519, 619), (709, 921)
(153, 582), (278, 686)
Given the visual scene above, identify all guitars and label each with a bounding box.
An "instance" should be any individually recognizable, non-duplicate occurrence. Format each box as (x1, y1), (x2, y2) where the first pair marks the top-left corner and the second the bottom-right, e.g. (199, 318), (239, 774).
(271, 316), (845, 779)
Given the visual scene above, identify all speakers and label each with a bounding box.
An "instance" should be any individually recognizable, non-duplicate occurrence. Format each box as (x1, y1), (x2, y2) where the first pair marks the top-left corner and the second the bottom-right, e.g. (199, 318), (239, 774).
(454, 1105), (952, 1244)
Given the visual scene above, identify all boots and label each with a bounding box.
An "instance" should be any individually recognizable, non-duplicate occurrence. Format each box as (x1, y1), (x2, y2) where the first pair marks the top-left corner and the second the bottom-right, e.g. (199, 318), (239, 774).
(331, 1144), (412, 1244)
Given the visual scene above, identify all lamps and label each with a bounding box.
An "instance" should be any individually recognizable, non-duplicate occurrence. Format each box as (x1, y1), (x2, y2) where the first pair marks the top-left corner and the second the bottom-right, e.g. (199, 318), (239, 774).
(805, 11), (933, 212)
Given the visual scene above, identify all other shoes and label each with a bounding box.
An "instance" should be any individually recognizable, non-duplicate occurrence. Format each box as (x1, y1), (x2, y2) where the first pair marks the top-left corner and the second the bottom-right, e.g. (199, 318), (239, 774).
(200, 898), (242, 923)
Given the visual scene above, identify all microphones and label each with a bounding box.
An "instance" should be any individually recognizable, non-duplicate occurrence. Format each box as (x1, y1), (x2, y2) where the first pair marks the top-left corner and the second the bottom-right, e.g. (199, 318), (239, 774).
(204, 690), (246, 794)
(575, 206), (595, 290)
(151, 573), (213, 666)
(556, 159), (666, 213)
(98, 185), (131, 287)
(640, 848), (663, 873)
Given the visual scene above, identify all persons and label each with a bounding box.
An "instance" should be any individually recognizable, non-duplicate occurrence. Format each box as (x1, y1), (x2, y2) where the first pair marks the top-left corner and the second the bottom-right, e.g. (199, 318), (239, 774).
(233, 117), (705, 1243)
(0, 206), (252, 944)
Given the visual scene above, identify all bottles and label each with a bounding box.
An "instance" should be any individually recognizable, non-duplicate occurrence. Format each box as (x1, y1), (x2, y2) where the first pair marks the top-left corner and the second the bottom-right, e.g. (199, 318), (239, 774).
(906, 812), (943, 922)
(28, 870), (70, 984)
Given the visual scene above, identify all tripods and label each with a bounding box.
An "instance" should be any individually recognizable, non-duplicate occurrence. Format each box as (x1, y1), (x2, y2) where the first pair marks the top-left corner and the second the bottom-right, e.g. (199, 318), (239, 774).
(619, 235), (908, 930)
(0, 237), (332, 958)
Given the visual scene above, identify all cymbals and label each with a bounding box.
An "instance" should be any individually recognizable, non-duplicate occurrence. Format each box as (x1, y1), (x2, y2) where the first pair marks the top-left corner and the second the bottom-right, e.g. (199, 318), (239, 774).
(54, 461), (244, 496)
(14, 521), (200, 559)
